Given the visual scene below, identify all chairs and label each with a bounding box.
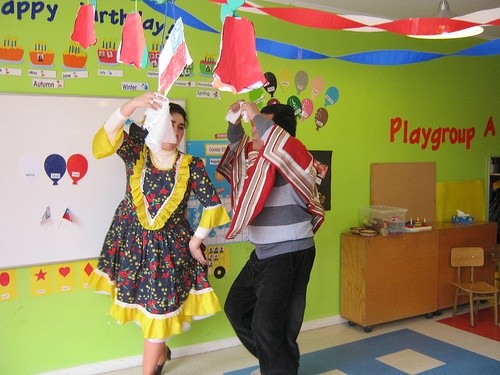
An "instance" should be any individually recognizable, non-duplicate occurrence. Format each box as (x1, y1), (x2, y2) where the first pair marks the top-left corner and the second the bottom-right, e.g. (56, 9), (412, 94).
(451, 247), (500, 327)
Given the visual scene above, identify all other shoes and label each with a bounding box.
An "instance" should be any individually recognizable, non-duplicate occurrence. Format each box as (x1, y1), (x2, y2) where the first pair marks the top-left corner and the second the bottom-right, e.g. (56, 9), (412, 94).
(153, 346), (172, 375)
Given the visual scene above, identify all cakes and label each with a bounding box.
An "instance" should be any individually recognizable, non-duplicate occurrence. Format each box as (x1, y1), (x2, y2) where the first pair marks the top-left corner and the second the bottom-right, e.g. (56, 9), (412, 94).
(403, 220), (433, 231)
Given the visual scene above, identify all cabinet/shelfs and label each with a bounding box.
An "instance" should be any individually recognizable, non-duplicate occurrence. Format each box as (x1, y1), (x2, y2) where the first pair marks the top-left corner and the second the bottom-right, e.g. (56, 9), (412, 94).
(340, 219), (498, 332)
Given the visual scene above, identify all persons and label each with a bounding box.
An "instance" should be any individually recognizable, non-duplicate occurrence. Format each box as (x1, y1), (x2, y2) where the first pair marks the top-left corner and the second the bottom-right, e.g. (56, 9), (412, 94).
(91, 91), (231, 375)
(216, 99), (325, 375)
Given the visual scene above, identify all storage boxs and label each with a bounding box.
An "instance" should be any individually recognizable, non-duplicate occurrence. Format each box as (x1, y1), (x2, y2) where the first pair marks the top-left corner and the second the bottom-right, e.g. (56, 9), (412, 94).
(357, 206), (408, 234)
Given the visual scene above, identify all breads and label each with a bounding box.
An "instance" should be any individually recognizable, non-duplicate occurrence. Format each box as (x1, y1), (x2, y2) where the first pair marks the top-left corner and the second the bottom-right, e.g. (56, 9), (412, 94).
(360, 229), (377, 236)
(351, 227), (360, 234)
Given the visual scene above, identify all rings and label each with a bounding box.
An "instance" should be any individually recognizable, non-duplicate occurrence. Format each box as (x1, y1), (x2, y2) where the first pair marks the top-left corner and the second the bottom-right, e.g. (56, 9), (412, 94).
(150, 99), (154, 105)
